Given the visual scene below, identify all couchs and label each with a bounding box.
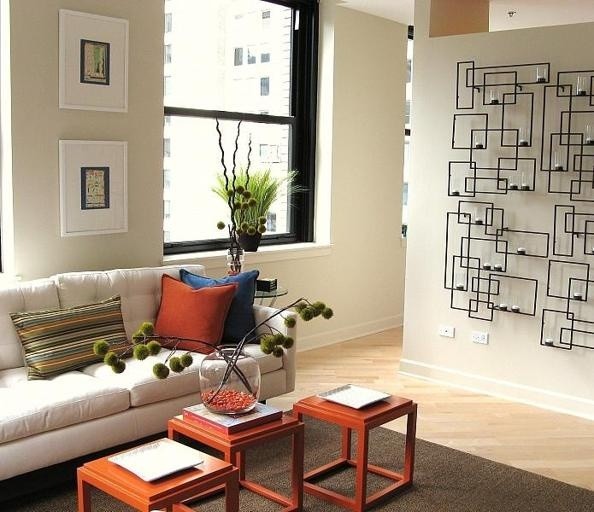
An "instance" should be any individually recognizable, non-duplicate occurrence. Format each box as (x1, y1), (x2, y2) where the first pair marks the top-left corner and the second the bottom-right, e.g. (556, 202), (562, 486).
(0, 264), (299, 500)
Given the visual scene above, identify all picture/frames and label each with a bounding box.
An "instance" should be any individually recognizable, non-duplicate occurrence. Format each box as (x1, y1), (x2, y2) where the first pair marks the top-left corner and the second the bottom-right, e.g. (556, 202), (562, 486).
(54, 6), (129, 239)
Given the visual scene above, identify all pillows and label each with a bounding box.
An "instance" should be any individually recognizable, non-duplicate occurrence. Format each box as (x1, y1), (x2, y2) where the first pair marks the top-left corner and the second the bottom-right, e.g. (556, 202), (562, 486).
(151, 268), (261, 355)
(6, 291), (133, 380)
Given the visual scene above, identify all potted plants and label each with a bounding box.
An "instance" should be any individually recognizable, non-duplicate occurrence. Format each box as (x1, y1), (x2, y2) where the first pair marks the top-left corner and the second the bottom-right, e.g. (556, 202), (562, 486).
(91, 294), (333, 416)
(210, 164), (313, 253)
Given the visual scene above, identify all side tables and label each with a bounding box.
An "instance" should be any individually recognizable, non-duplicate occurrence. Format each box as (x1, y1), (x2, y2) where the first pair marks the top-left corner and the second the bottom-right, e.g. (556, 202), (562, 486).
(253, 288), (287, 308)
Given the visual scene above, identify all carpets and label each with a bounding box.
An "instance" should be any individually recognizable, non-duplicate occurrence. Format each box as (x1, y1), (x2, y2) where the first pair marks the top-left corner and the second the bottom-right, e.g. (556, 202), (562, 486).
(0, 408), (593, 511)
